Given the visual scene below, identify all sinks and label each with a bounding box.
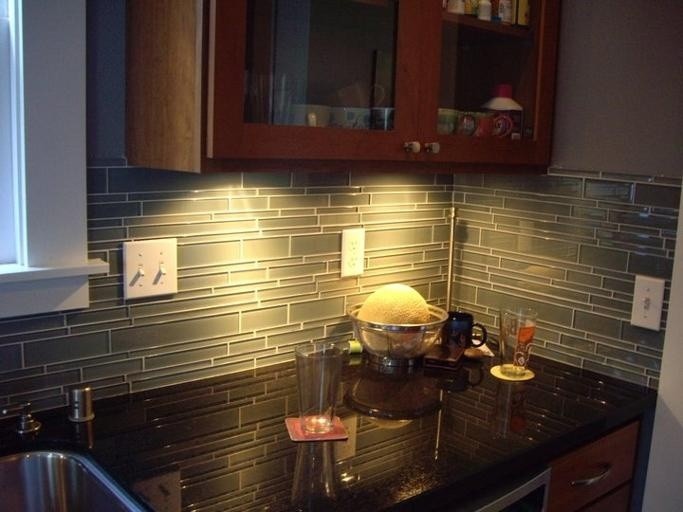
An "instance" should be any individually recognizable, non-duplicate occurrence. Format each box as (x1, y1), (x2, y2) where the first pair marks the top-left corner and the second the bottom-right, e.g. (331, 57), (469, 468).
(1, 450), (151, 511)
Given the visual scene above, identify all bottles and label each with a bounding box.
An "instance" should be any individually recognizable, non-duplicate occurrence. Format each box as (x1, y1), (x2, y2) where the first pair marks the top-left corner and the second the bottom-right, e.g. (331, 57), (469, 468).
(445, 0), (531, 30)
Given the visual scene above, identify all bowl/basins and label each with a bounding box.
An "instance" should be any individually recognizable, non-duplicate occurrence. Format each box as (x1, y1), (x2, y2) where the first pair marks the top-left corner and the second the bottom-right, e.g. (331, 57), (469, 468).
(343, 366), (445, 419)
(346, 303), (450, 366)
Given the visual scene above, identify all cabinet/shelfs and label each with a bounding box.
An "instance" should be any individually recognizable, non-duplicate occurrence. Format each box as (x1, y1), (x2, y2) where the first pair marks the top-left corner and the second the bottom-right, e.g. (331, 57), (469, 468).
(547, 392), (656, 512)
(125, 0), (561, 175)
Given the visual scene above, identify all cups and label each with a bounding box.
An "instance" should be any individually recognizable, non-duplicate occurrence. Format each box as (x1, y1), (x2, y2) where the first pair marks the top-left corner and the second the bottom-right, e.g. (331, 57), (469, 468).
(441, 311), (488, 351)
(288, 439), (341, 512)
(491, 376), (529, 443)
(498, 306), (537, 377)
(295, 342), (344, 434)
(289, 104), (394, 129)
(436, 360), (484, 393)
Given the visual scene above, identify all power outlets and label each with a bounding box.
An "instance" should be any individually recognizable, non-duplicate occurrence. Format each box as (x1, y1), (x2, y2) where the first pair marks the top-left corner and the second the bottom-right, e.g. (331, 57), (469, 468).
(341, 227), (364, 277)
